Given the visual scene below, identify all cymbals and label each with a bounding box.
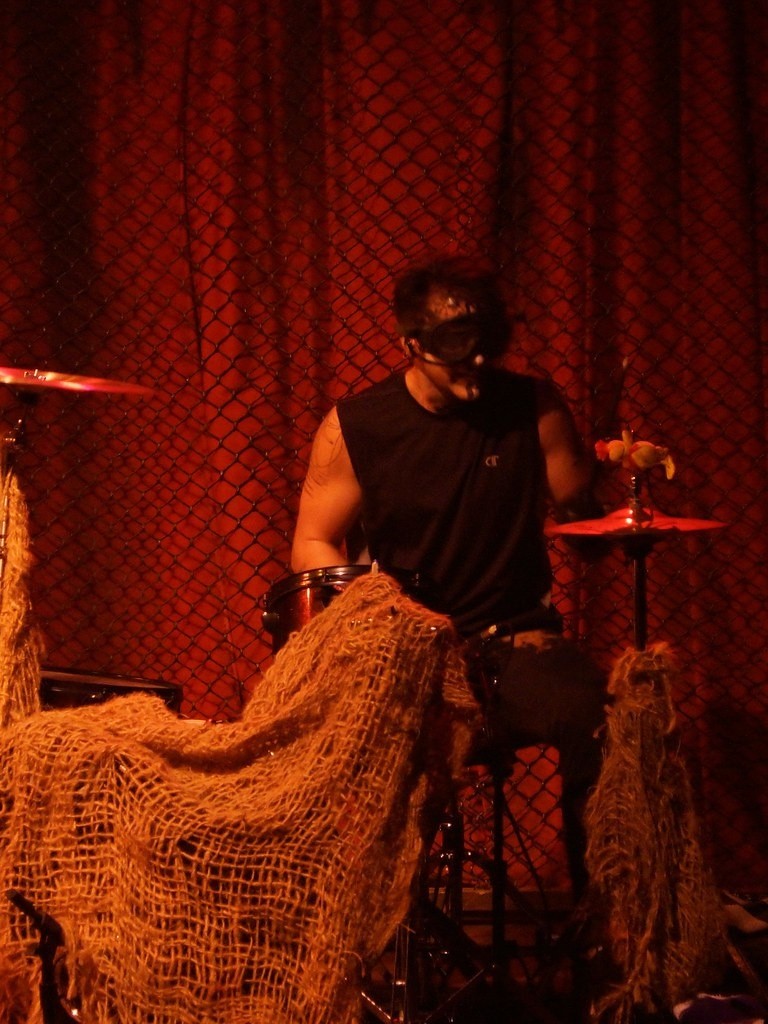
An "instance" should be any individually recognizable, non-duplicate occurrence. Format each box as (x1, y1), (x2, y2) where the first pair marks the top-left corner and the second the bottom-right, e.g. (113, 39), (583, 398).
(544, 509), (726, 543)
(0, 363), (158, 411)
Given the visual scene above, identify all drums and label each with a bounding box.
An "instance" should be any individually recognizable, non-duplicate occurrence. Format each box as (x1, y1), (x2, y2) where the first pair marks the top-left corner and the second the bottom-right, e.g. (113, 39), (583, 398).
(257, 564), (407, 670)
(27, 663), (182, 723)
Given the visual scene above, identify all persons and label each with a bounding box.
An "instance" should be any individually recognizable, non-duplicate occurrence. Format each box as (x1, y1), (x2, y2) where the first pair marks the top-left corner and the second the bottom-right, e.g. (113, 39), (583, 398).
(292, 254), (667, 901)
